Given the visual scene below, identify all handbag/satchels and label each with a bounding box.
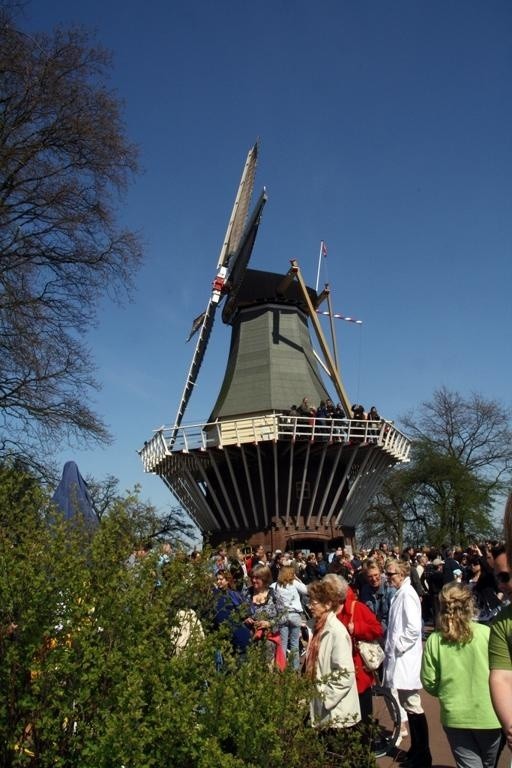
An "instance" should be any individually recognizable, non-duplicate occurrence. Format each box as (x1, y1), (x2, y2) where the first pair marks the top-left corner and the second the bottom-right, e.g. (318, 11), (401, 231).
(355, 639), (386, 671)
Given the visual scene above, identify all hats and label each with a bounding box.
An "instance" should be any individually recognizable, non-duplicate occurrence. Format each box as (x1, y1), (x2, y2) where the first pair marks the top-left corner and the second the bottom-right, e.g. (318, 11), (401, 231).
(453, 569), (463, 575)
(432, 559), (445, 565)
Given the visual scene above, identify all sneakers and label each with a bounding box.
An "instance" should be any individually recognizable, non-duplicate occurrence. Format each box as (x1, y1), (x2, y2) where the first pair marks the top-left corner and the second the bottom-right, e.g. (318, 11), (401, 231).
(385, 728), (408, 748)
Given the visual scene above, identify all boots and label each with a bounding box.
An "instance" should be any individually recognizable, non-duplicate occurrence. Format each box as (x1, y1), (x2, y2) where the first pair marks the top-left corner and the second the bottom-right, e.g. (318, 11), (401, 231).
(400, 713), (433, 766)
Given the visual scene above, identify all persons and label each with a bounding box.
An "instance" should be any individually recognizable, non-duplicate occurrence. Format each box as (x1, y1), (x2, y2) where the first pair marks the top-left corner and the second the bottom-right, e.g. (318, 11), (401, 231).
(285, 397), (408, 449)
(5, 537), (512, 767)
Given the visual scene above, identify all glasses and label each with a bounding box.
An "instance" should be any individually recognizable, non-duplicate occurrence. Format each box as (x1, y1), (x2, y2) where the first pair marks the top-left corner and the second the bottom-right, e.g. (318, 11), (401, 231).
(385, 571), (398, 576)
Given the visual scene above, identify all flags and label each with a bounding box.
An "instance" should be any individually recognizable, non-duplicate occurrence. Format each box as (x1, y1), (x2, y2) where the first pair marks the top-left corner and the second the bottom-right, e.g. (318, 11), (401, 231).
(323, 241), (327, 256)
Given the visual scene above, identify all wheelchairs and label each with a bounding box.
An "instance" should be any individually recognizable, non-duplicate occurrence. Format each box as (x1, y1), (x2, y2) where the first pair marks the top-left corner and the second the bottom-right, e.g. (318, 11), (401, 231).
(367, 669), (401, 761)
